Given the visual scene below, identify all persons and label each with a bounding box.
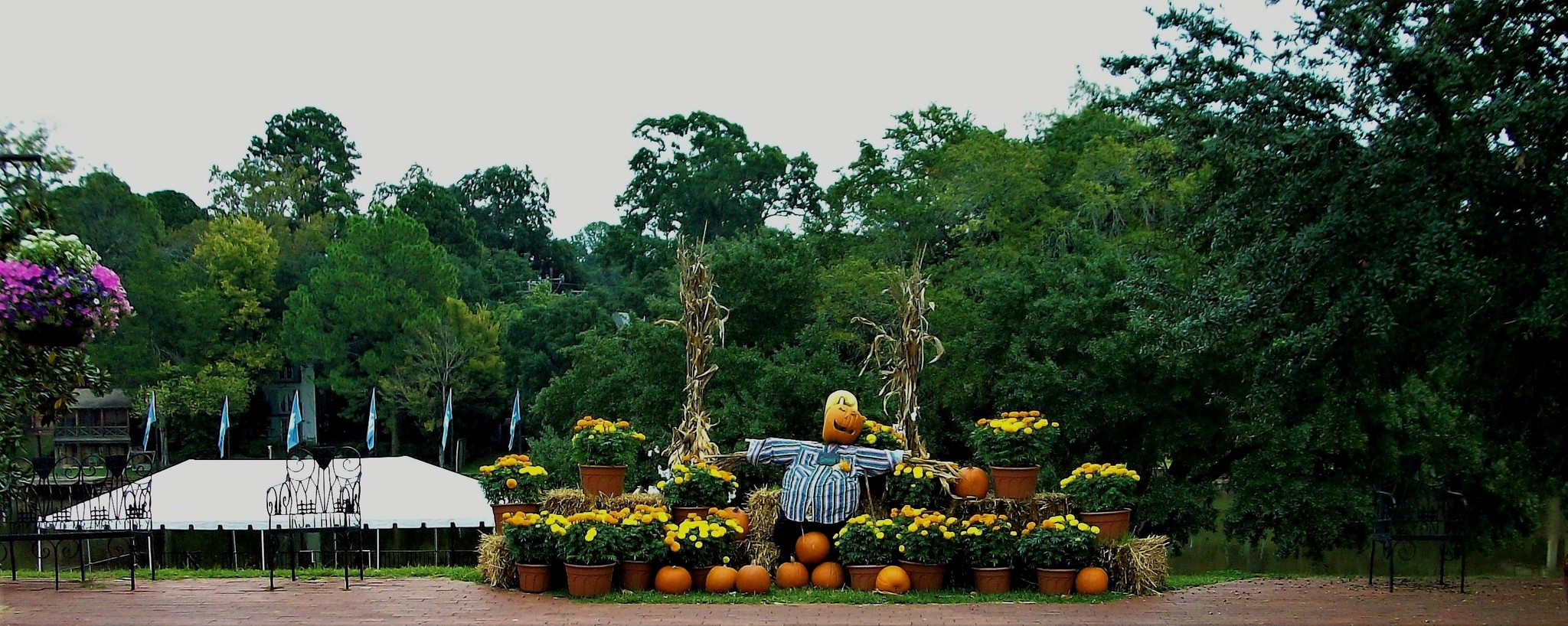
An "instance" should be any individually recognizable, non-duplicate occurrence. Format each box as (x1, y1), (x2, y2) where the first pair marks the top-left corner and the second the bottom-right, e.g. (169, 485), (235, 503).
(707, 402), (961, 579)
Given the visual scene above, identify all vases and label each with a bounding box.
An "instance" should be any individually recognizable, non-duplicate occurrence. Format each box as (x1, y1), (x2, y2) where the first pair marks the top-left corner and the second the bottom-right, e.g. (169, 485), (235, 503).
(1037, 568), (1075, 595)
(16, 298), (89, 347)
(1080, 508), (1131, 543)
(621, 561), (654, 591)
(491, 502), (543, 535)
(686, 565), (720, 591)
(847, 565), (887, 591)
(896, 558), (945, 591)
(671, 504), (709, 527)
(971, 566), (1011, 594)
(514, 558), (616, 598)
(989, 463), (1041, 500)
(577, 463), (629, 498)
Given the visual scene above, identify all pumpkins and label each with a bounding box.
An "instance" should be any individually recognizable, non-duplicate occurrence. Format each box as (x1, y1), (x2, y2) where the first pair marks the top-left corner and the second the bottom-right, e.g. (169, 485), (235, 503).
(811, 561), (845, 589)
(735, 559), (771, 593)
(795, 530), (830, 563)
(1075, 566), (1108, 594)
(721, 506), (749, 539)
(654, 565), (692, 594)
(875, 565), (910, 594)
(953, 463), (990, 499)
(705, 564), (738, 592)
(775, 555), (809, 589)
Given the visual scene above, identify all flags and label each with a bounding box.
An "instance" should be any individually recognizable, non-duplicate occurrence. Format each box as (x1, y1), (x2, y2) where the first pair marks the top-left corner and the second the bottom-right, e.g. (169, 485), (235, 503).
(508, 390), (521, 451)
(286, 390), (302, 452)
(365, 388), (377, 450)
(142, 390), (156, 452)
(441, 389), (453, 452)
(218, 396), (230, 458)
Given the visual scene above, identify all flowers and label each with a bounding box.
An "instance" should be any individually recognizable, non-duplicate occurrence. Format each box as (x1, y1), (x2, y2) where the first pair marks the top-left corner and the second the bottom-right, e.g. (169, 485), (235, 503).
(478, 416), (744, 567)
(831, 409), (1140, 570)
(0, 229), (137, 349)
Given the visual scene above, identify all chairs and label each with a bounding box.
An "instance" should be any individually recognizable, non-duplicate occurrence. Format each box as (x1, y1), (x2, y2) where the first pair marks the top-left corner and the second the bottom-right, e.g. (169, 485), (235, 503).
(0, 453), (155, 591)
(1367, 438), (1469, 595)
(267, 444), (364, 591)
